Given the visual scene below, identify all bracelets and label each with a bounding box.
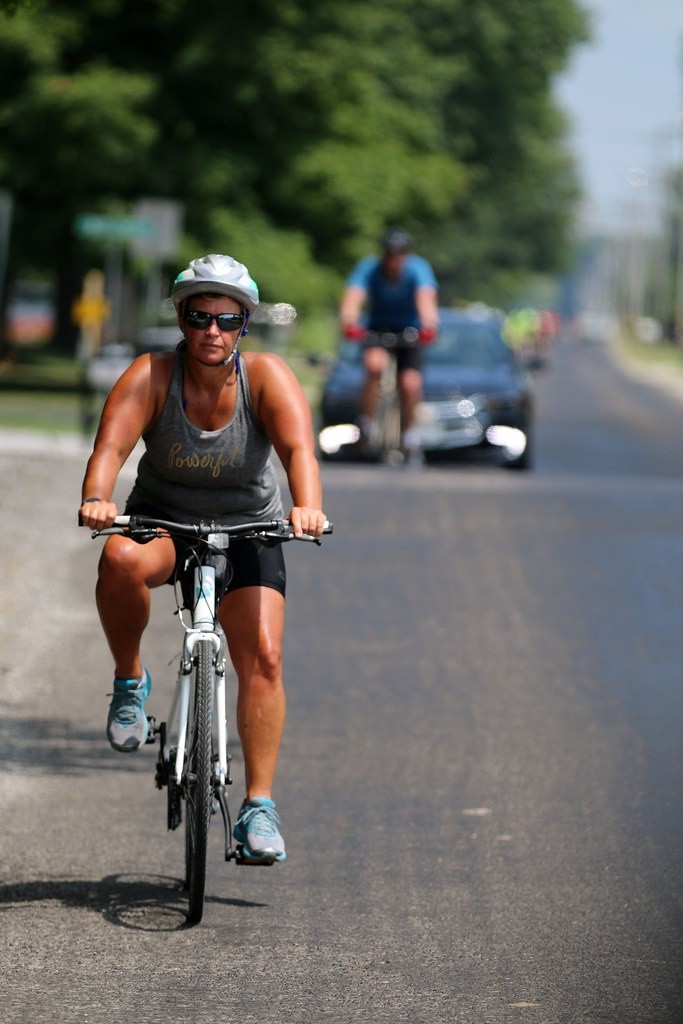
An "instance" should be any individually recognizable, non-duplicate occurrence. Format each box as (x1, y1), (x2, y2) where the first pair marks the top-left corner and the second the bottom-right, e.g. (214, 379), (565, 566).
(80, 497), (103, 505)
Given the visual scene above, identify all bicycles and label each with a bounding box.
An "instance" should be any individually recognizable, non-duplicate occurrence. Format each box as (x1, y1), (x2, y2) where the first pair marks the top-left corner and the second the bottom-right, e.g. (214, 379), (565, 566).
(355, 322), (425, 478)
(76, 513), (333, 925)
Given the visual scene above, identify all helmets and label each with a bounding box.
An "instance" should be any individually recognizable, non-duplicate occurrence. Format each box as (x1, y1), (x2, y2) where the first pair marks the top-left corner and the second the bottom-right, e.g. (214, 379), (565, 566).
(171, 253), (260, 319)
(379, 229), (412, 248)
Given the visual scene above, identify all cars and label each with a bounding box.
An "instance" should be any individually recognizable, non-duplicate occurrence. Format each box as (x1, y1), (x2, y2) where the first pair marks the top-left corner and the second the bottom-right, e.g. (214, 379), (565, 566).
(319, 305), (543, 473)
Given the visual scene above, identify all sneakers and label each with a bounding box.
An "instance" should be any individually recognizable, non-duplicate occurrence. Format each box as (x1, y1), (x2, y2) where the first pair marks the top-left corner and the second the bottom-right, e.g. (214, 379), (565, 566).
(233, 797), (287, 863)
(106, 666), (152, 753)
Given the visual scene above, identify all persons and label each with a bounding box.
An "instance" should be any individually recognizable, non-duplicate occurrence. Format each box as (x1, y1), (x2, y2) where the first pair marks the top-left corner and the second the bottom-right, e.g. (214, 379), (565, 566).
(78, 253), (326, 863)
(342, 230), (441, 457)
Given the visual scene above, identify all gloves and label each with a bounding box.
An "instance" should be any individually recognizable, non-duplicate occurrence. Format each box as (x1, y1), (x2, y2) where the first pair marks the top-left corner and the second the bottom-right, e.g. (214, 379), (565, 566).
(344, 323), (368, 342)
(418, 326), (434, 345)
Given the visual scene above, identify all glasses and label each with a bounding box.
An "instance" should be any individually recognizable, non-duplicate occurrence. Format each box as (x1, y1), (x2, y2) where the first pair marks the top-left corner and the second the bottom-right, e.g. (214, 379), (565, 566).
(180, 310), (245, 332)
